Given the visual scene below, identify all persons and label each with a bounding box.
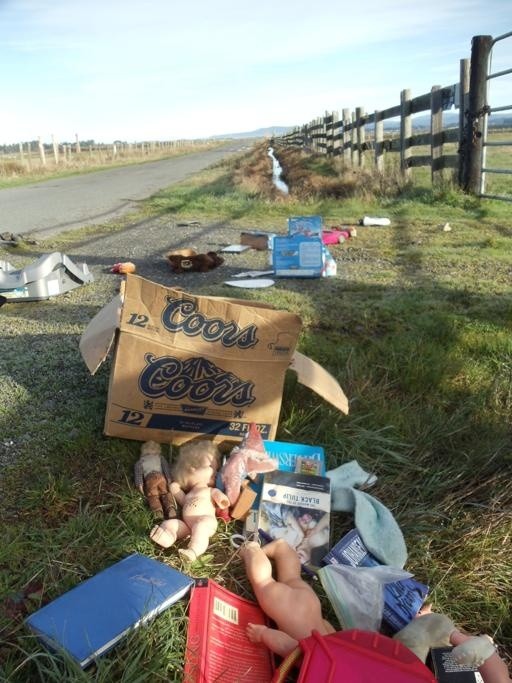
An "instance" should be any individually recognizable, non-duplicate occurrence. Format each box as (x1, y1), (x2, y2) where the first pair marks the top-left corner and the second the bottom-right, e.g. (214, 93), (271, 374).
(241, 539), (341, 667)
(135, 440), (178, 520)
(150, 440), (229, 558)
(393, 608), (511, 683)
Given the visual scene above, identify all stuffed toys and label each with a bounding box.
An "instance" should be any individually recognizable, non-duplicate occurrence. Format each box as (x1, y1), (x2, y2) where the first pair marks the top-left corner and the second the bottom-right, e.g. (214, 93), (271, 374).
(167, 248), (226, 275)
(108, 260), (137, 274)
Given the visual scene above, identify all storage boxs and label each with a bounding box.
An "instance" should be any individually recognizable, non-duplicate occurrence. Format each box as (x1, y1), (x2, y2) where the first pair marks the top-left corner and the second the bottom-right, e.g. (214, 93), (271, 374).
(241, 229), (274, 251)
(273, 235), (324, 281)
(287, 216), (324, 238)
(76, 272), (349, 445)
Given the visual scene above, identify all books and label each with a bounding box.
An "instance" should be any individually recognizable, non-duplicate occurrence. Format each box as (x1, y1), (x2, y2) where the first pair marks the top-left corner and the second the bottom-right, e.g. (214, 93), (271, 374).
(185, 579), (280, 682)
(24, 551), (194, 671)
(242, 438), (327, 543)
(426, 645), (483, 683)
(320, 527), (430, 635)
(255, 469), (330, 576)
